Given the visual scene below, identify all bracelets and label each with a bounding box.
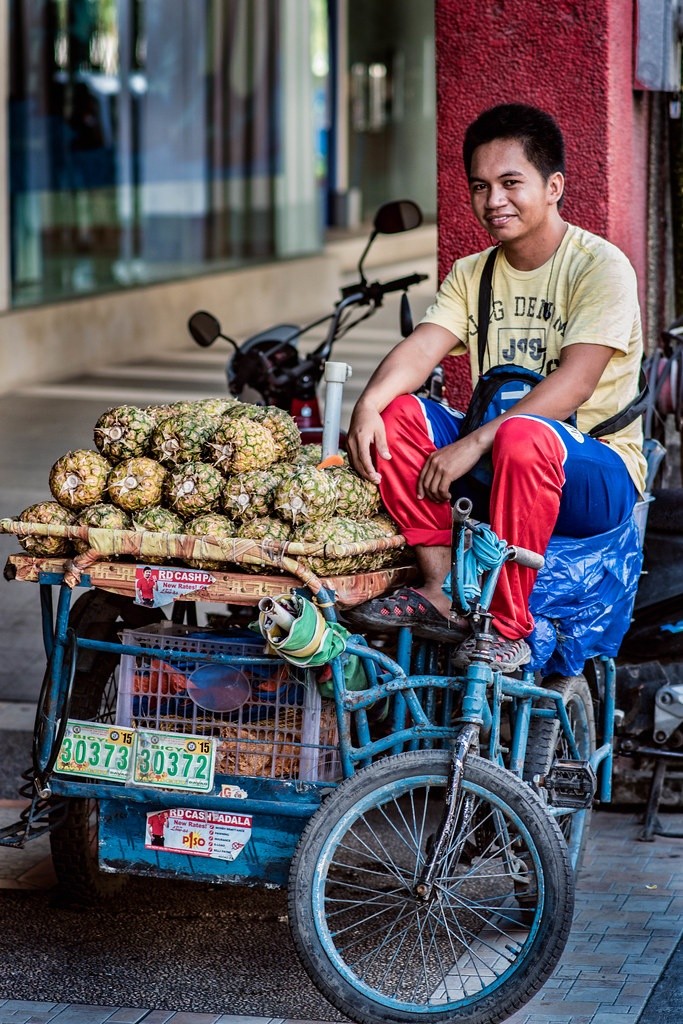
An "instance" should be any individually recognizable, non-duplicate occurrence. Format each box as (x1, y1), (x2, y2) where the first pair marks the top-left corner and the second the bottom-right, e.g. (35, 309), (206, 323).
(155, 581), (157, 582)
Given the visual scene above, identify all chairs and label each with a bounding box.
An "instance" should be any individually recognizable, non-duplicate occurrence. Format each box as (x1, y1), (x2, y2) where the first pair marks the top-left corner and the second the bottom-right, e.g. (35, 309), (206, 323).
(476, 440), (665, 664)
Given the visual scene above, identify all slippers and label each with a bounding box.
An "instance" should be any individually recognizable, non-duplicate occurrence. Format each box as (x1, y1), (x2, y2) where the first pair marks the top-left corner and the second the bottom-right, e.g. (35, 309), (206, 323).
(340, 587), (467, 644)
(455, 633), (531, 672)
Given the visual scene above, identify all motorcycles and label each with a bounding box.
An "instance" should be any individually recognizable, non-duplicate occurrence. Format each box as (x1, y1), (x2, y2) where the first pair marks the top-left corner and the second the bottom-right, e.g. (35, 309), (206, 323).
(190, 203), (649, 843)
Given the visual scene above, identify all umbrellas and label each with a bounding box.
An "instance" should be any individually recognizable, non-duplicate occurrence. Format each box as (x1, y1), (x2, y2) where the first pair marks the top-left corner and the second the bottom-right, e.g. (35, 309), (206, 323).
(257, 593), (391, 721)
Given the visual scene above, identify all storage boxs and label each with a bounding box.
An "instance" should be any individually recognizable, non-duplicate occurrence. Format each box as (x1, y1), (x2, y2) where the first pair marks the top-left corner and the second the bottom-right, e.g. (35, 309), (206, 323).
(114, 623), (346, 785)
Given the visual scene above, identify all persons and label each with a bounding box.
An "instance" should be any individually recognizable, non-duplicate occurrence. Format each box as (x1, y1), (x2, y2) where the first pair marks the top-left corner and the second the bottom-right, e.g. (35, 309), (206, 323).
(347, 104), (648, 673)
(147, 813), (166, 846)
(137, 567), (158, 607)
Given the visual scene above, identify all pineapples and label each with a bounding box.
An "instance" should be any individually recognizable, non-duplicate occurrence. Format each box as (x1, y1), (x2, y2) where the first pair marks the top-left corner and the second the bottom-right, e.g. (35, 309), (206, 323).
(14, 397), (412, 576)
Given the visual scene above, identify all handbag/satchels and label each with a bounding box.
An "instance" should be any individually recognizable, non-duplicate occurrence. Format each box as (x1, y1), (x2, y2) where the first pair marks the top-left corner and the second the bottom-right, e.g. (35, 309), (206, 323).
(461, 364), (578, 489)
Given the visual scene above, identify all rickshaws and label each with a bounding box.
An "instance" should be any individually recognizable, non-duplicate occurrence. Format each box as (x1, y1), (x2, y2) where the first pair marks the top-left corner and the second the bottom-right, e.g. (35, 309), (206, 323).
(0, 198), (645, 1024)
